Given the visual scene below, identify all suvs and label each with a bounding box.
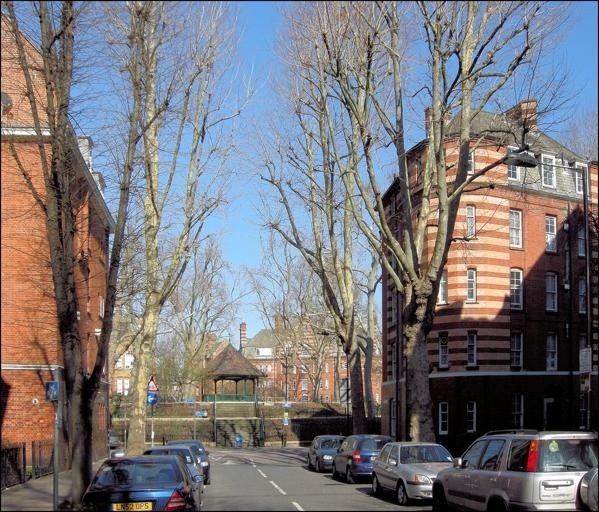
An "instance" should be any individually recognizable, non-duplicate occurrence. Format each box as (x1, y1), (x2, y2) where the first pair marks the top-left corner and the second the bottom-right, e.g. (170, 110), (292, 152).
(432, 428), (599, 511)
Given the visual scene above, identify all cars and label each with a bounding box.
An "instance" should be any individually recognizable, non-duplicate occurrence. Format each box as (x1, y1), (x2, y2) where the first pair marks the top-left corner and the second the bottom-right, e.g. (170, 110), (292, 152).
(371, 442), (455, 505)
(81, 440), (211, 511)
(307, 435), (346, 472)
(331, 435), (394, 484)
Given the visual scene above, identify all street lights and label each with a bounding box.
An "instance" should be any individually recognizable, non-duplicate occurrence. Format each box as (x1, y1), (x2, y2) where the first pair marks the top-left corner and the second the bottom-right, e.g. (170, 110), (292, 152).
(316, 331), (350, 424)
(502, 153), (594, 426)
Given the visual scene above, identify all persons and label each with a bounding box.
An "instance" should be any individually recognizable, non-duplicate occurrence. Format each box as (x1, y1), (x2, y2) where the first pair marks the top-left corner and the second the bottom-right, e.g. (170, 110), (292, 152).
(223, 431), (229, 447)
(282, 430), (288, 448)
(234, 432), (243, 449)
(252, 431), (260, 447)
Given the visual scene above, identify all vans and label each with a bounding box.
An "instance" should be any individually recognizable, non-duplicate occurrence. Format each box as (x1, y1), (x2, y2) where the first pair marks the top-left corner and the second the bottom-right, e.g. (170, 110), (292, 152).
(107, 430), (120, 447)
